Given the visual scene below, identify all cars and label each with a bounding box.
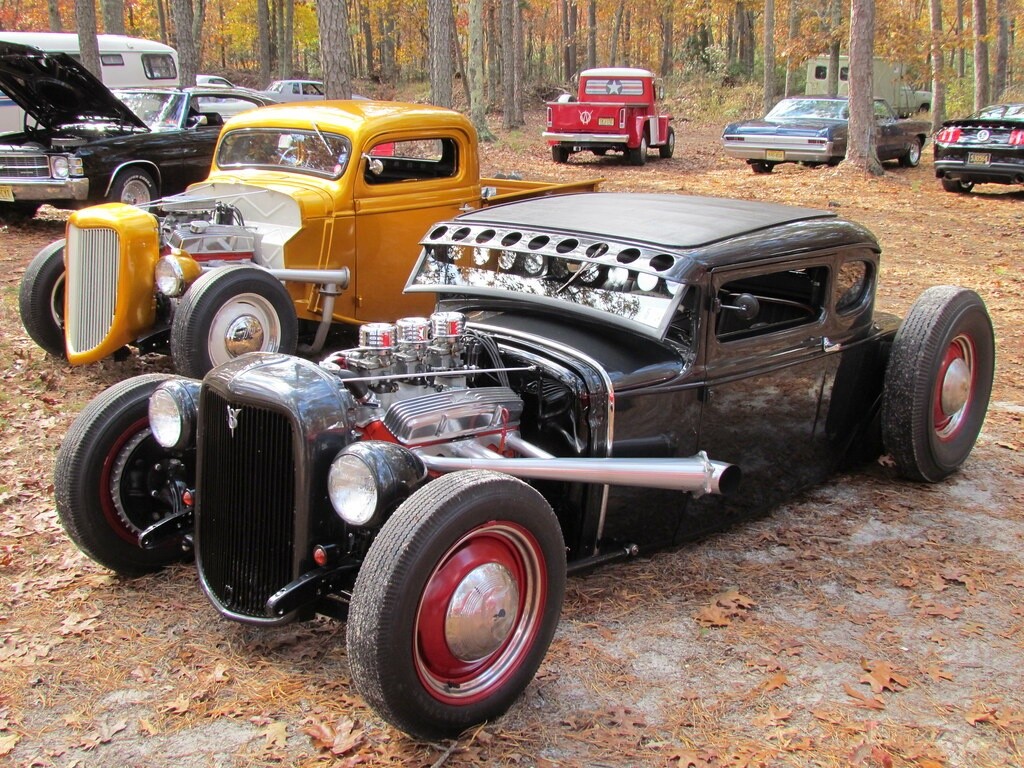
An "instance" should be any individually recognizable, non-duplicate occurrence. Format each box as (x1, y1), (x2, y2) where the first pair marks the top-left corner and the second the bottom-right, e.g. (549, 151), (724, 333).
(0, 37), (345, 226)
(933, 102), (1024, 194)
(193, 73), (373, 124)
(721, 95), (933, 173)
(51, 189), (997, 744)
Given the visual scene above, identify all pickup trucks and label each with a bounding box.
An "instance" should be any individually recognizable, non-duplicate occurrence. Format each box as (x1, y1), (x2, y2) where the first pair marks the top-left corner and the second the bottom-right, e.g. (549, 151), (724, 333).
(18, 98), (608, 383)
(541, 67), (675, 165)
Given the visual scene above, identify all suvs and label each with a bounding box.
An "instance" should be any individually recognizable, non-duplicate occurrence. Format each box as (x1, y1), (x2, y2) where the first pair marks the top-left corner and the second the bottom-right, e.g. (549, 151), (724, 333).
(803, 54), (932, 117)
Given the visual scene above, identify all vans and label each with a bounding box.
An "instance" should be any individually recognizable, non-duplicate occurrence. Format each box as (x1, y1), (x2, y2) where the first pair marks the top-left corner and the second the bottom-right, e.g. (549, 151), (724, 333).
(0, 31), (181, 138)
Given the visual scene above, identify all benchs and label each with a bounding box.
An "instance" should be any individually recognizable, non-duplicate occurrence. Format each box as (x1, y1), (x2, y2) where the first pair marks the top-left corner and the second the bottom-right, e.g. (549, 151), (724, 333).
(144, 105), (224, 126)
